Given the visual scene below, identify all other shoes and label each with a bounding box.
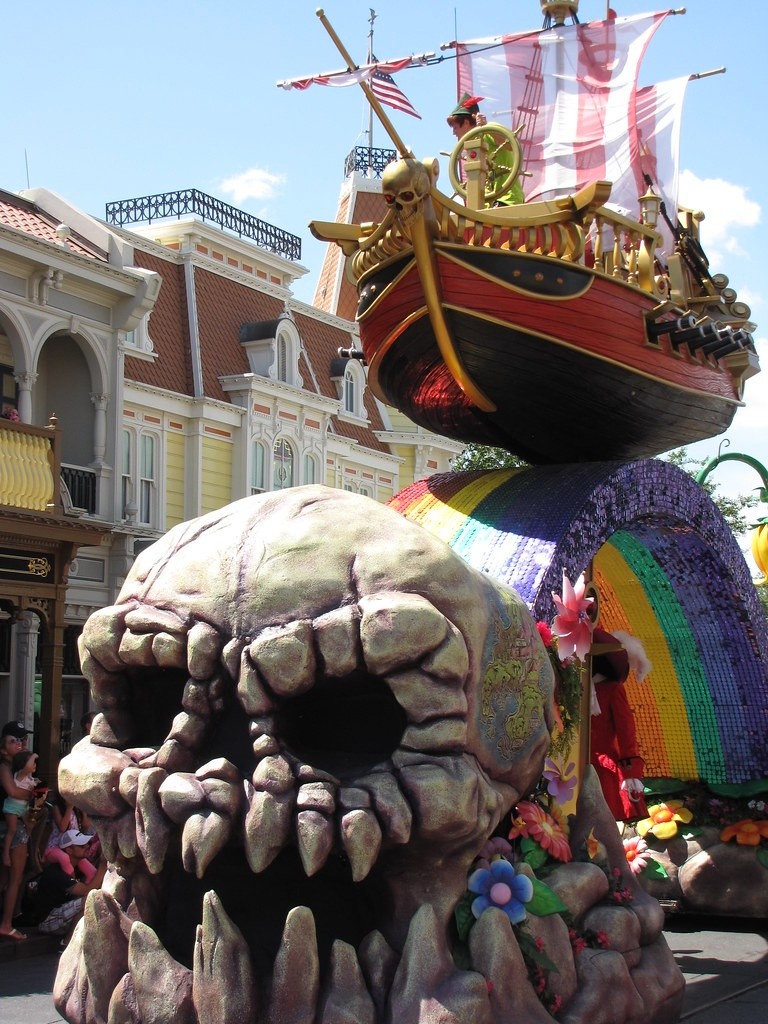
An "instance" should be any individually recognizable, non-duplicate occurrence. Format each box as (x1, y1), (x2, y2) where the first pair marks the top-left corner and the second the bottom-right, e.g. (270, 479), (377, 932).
(59, 939), (67, 953)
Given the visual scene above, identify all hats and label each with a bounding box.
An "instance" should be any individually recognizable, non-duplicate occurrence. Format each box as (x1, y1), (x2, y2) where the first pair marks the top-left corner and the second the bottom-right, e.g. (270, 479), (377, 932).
(59, 830), (94, 850)
(3, 721), (34, 738)
(449, 92), (484, 115)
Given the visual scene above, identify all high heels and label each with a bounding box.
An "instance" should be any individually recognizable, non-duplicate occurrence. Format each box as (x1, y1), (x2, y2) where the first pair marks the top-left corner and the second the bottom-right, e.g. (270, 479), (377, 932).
(0, 928), (29, 942)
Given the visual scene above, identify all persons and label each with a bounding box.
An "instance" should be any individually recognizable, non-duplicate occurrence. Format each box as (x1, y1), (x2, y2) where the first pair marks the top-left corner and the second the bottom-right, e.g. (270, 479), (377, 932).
(8, 408), (21, 422)
(445, 93), (524, 209)
(0, 712), (108, 946)
(588, 680), (649, 823)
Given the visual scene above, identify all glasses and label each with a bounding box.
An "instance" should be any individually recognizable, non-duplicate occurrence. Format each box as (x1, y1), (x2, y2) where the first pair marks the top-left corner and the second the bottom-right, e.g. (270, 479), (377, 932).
(20, 735), (28, 742)
(7, 738), (20, 746)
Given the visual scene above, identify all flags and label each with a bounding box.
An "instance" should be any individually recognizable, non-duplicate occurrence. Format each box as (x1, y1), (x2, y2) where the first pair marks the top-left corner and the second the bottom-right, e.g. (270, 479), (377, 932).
(366, 53), (422, 120)
